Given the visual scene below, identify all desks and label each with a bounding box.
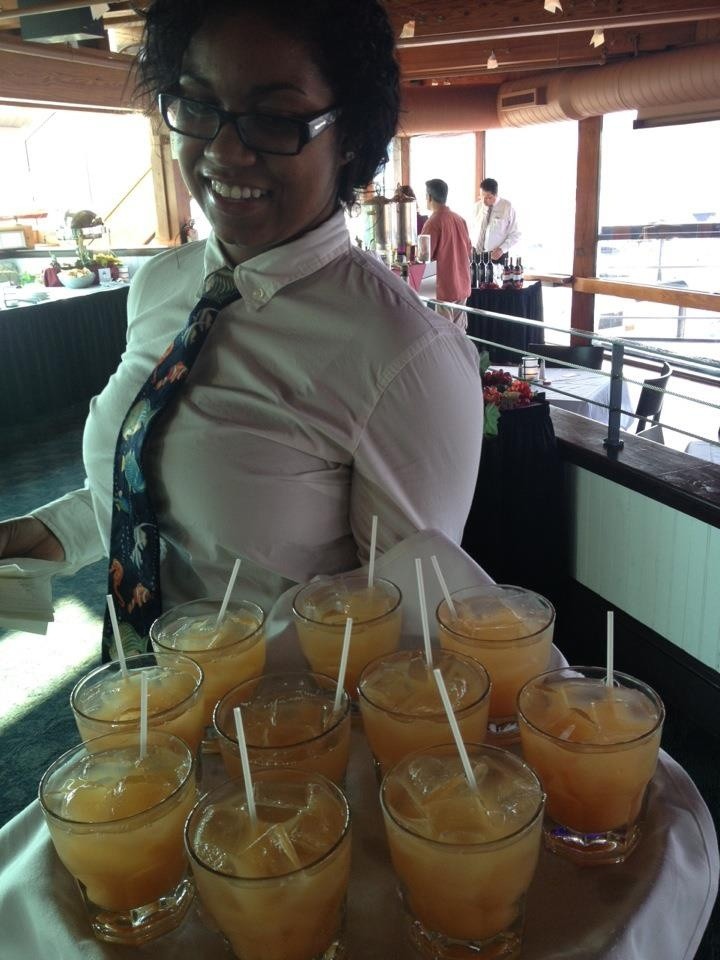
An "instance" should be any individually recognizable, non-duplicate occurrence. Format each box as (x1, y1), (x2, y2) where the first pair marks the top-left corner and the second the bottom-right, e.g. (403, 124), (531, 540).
(461, 394), (575, 598)
(482, 362), (637, 433)
(468, 277), (545, 366)
(0, 277), (131, 428)
(685, 440), (720, 468)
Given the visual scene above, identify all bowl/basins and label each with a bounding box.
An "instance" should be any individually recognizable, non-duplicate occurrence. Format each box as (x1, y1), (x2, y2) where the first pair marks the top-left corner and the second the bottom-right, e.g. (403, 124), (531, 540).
(55, 269), (96, 288)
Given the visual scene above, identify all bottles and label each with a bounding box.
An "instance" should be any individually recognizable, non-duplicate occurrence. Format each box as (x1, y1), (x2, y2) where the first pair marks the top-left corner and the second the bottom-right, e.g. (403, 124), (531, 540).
(469, 246), (524, 290)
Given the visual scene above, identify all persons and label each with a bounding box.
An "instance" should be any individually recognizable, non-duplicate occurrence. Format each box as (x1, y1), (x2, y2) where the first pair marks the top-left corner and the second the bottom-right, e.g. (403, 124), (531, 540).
(406, 177), (473, 336)
(469, 178), (521, 267)
(0, 0), (485, 677)
(392, 185), (428, 255)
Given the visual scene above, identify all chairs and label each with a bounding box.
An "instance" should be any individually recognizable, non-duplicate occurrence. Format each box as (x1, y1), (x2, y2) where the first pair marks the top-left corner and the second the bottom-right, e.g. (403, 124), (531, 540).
(526, 343), (605, 371)
(633, 361), (674, 434)
(636, 423), (664, 447)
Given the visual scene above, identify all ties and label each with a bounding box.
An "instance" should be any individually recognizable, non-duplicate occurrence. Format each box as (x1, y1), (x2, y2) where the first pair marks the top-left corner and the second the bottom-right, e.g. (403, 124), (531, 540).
(93, 267), (246, 675)
(475, 204), (494, 256)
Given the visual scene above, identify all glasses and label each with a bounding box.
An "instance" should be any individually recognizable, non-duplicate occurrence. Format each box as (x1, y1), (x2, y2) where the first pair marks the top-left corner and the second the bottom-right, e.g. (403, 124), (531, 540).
(152, 83), (353, 159)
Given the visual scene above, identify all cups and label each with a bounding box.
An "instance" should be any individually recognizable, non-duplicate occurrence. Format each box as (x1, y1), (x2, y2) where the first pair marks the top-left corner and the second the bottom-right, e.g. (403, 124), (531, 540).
(38, 571), (666, 960)
